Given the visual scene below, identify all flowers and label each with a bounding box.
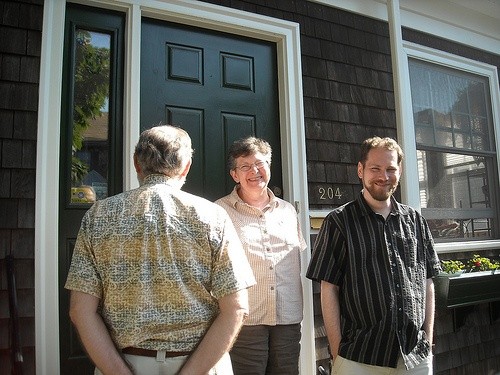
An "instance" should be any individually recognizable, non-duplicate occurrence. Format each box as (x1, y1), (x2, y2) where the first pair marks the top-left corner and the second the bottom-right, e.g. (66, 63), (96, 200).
(442, 256), (499, 273)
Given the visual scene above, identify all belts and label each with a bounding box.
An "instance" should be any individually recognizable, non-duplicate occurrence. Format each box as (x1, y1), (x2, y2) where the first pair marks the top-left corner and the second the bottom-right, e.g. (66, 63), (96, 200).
(121, 347), (193, 358)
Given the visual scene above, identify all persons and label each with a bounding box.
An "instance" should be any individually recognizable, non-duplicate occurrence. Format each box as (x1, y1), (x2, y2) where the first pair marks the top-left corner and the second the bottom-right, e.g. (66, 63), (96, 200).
(210, 136), (308, 375)
(306, 136), (442, 375)
(64, 124), (258, 375)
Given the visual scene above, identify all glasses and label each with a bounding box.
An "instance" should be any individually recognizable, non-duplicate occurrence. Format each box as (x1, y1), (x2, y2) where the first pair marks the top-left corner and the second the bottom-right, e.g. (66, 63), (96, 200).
(236, 160), (267, 171)
